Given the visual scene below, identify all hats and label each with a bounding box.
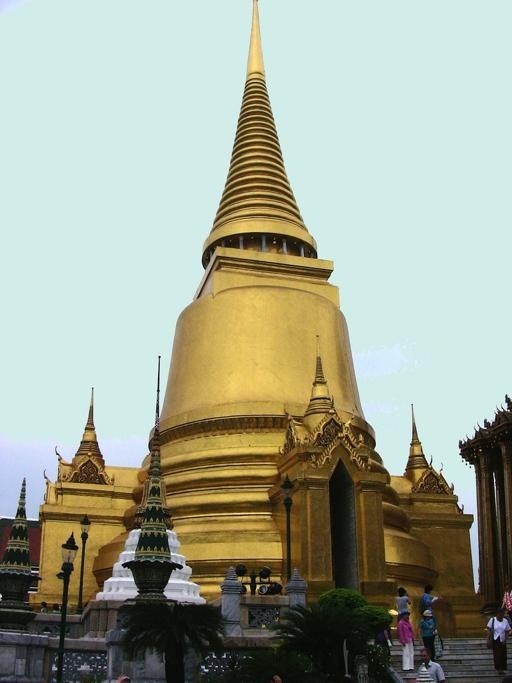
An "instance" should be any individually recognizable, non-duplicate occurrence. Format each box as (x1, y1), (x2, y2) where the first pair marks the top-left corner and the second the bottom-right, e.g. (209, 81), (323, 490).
(423, 610), (433, 617)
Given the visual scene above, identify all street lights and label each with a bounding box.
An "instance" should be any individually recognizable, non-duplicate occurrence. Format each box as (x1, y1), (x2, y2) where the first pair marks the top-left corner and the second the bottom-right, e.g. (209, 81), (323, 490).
(55, 532), (81, 678)
(281, 473), (295, 582)
(74, 512), (92, 617)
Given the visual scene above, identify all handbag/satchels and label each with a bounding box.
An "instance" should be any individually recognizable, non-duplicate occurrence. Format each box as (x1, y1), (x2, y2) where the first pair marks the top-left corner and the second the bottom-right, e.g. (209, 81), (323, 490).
(487, 617), (495, 649)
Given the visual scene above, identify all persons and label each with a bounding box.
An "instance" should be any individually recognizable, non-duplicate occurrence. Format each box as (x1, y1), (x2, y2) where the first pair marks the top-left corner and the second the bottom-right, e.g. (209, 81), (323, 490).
(418, 608), (439, 661)
(419, 583), (439, 622)
(392, 585), (412, 624)
(38, 600), (47, 613)
(414, 647), (446, 683)
(395, 607), (417, 673)
(377, 615), (393, 656)
(49, 601), (60, 613)
(498, 581), (512, 620)
(483, 607), (512, 676)
